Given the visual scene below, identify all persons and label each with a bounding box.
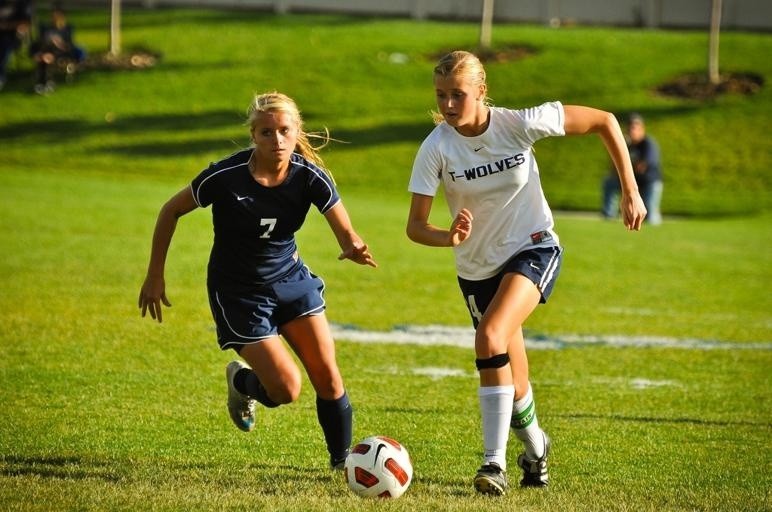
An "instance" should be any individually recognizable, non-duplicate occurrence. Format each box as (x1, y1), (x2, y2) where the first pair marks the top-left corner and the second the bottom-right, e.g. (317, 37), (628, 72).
(137, 92), (379, 472)
(405, 50), (648, 497)
(597, 113), (665, 226)
(0, 0), (34, 93)
(27, 7), (87, 96)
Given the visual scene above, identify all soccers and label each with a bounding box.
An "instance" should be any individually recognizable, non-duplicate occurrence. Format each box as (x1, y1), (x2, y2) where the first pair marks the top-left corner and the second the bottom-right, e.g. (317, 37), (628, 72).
(345, 437), (412, 500)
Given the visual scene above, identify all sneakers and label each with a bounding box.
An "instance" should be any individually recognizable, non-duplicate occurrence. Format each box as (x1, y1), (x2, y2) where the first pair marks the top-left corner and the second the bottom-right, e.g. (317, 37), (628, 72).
(518, 428), (550, 488)
(227, 361), (257, 432)
(473, 462), (507, 496)
(327, 449), (349, 472)
